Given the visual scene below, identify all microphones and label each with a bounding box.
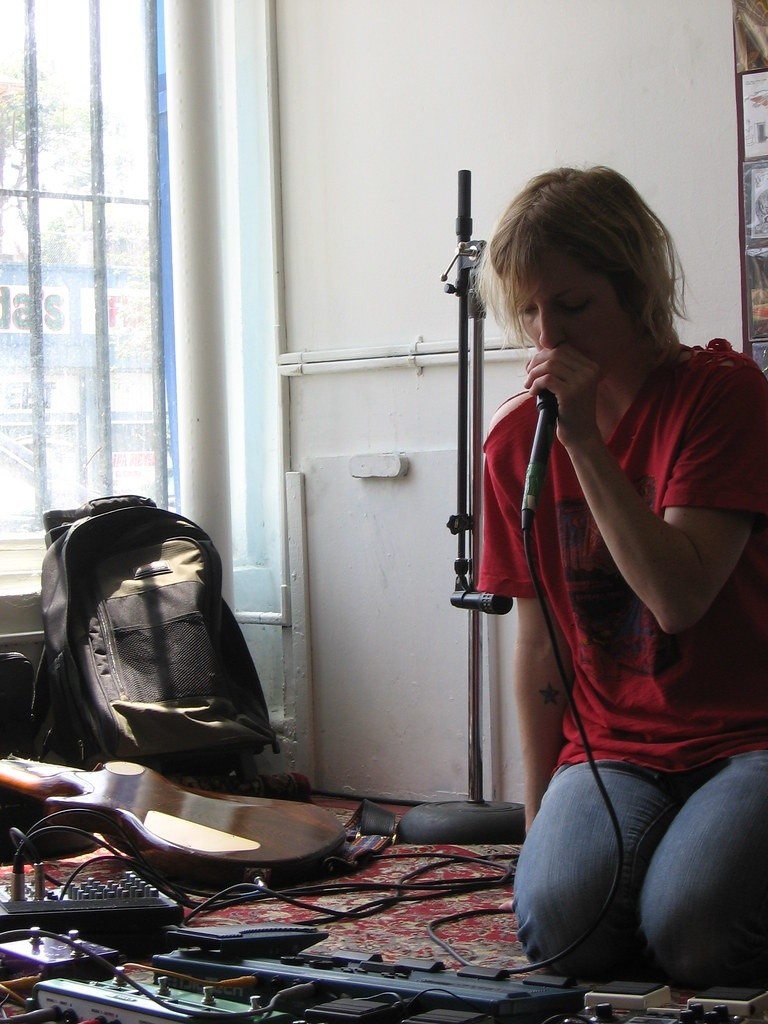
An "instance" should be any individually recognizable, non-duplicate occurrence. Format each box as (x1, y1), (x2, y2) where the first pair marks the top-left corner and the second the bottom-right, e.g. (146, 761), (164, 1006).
(522, 391), (559, 530)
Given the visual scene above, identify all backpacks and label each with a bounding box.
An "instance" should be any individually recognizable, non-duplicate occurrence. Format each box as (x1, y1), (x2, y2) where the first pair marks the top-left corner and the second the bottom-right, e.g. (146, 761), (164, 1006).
(29, 494), (280, 766)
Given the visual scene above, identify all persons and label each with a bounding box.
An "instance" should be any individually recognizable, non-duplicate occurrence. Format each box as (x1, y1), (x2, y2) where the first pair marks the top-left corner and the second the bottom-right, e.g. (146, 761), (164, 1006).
(475, 163), (768, 988)
